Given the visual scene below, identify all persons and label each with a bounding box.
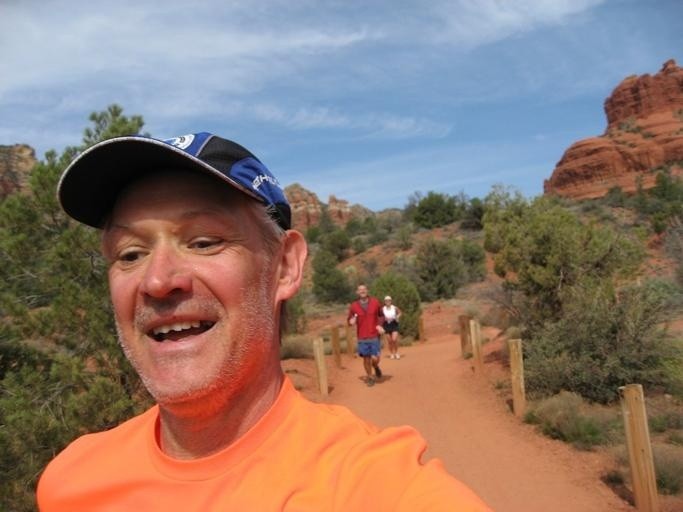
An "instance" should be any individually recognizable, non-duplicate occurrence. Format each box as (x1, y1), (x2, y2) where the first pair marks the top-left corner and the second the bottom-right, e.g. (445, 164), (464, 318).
(33, 128), (494, 512)
(344, 280), (385, 386)
(379, 294), (401, 360)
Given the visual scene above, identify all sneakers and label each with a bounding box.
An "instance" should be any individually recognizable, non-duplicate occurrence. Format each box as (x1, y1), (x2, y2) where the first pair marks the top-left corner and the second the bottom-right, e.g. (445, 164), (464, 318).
(367, 375), (375, 386)
(375, 366), (382, 377)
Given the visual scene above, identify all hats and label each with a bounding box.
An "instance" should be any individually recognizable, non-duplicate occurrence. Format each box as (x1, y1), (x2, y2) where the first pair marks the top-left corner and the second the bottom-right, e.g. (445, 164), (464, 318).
(384, 295), (392, 300)
(56, 132), (293, 229)
